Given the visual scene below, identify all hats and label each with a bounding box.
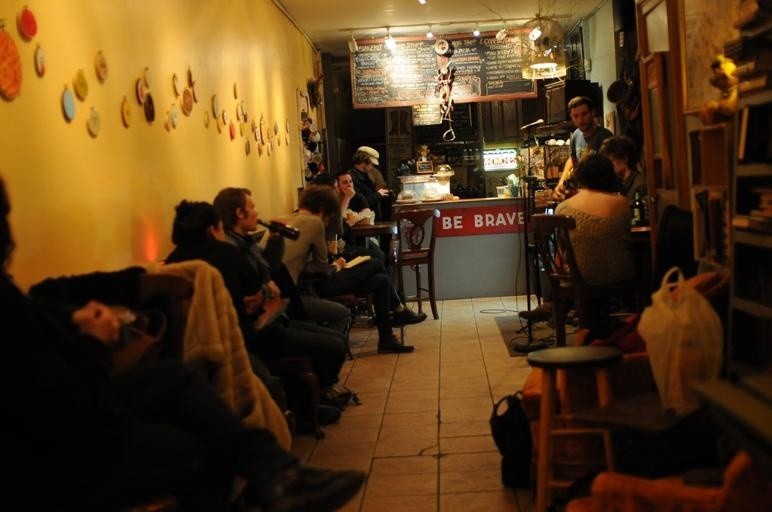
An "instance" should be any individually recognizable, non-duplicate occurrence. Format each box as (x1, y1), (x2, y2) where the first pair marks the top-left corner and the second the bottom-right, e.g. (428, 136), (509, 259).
(359, 146), (379, 165)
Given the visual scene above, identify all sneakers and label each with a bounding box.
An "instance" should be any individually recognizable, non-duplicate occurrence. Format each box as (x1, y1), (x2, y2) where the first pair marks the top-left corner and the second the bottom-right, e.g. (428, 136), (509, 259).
(394, 307), (426, 327)
(519, 303), (567, 329)
(317, 384), (351, 424)
(379, 342), (414, 353)
(260, 466), (366, 511)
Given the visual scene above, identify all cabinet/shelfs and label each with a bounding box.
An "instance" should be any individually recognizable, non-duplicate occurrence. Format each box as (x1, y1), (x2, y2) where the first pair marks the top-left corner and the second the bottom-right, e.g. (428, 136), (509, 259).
(521, 125), (576, 307)
(688, 83), (772, 471)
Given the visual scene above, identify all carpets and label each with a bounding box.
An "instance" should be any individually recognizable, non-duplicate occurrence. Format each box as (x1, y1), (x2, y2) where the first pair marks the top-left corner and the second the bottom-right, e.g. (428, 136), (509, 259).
(494, 315), (576, 357)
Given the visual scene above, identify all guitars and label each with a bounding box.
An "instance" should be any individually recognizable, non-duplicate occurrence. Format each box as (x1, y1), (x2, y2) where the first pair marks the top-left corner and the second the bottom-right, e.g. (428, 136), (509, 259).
(552, 131), (578, 204)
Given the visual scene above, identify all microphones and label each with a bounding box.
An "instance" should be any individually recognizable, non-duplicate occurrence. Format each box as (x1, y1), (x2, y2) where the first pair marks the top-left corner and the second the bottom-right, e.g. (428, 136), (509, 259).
(520, 118), (544, 131)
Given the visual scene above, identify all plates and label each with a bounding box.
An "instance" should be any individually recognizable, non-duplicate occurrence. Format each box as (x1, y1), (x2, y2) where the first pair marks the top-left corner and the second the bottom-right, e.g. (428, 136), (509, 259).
(395, 199), (417, 203)
(420, 197), (442, 202)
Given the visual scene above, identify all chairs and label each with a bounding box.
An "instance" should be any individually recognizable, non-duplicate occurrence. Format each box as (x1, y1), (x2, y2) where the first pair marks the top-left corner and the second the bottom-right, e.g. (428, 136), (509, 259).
(530, 213), (647, 345)
(391, 208), (439, 319)
(296, 371), (326, 441)
(331, 293), (375, 328)
(344, 222), (399, 287)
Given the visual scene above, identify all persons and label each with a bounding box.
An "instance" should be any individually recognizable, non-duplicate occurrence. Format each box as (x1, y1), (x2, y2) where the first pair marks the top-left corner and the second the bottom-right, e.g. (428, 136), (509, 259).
(165, 200), (348, 425)
(519, 136), (646, 322)
(566, 95), (613, 168)
(1, 177), (367, 512)
(213, 144), (426, 408)
(553, 154), (637, 337)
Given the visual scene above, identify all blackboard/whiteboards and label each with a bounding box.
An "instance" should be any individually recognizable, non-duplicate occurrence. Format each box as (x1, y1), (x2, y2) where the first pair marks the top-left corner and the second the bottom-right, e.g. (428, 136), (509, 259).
(348, 26), (538, 110)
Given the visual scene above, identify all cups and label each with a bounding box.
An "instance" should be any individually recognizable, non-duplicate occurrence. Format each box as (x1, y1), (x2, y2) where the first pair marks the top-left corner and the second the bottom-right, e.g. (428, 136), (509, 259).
(496, 184), (508, 198)
(401, 190), (414, 200)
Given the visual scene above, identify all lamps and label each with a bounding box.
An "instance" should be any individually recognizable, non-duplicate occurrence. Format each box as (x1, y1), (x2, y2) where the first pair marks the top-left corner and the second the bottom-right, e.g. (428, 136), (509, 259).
(349, 17), (508, 53)
(519, 1), (567, 80)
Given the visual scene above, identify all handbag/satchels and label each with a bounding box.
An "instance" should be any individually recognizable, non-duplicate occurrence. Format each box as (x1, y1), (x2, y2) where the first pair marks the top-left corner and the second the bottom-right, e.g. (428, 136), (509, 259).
(489, 392), (531, 489)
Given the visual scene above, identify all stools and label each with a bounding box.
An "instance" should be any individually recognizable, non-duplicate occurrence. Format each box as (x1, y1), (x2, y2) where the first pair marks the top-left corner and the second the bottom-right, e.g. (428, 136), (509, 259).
(527, 346), (619, 512)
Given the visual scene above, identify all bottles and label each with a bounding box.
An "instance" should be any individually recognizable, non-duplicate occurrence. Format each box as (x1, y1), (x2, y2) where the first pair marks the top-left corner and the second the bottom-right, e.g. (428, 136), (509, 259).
(633, 191), (645, 226)
(256, 217), (301, 240)
